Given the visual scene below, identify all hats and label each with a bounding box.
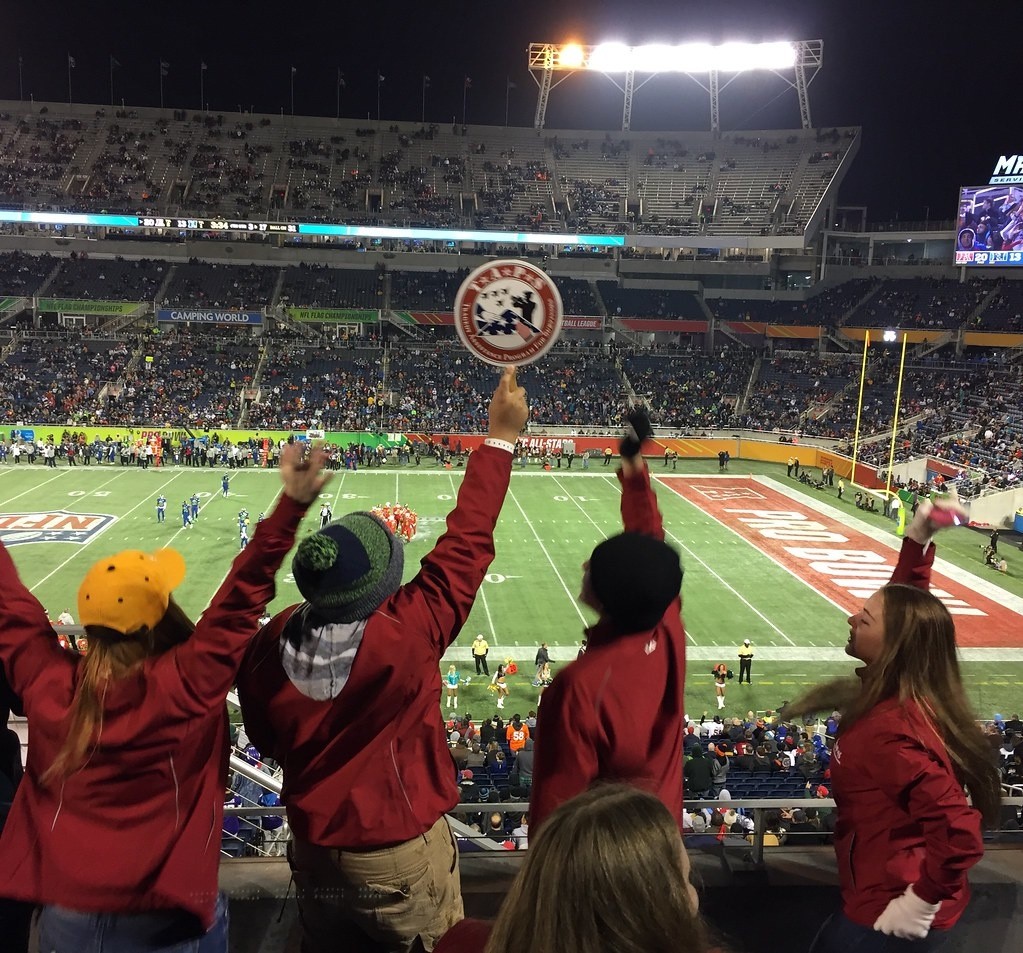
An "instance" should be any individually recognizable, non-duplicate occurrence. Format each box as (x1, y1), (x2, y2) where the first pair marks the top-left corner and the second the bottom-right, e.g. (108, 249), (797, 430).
(461, 769), (474, 779)
(292, 511), (406, 624)
(744, 638), (750, 645)
(476, 634), (483, 640)
(78, 548), (185, 641)
(588, 531), (684, 629)
(832, 711), (842, 717)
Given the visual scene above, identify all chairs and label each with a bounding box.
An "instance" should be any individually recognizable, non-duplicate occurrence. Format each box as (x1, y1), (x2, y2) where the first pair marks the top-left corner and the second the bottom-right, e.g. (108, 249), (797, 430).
(457, 740), (515, 789)
(679, 738), (839, 800)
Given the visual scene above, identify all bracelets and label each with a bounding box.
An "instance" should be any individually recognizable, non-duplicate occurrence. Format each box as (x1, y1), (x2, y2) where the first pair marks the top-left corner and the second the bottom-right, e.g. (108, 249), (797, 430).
(484, 438), (515, 453)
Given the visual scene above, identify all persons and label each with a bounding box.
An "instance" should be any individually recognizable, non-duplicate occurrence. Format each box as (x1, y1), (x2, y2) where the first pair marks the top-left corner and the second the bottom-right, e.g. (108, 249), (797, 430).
(444, 711), (537, 853)
(982, 713), (1022, 841)
(683, 700), (842, 847)
(0, 327), (1023, 572)
(220, 723), (293, 857)
(0, 440), (335, 953)
(236, 365), (528, 953)
(44, 608), (88, 657)
(486, 785), (710, 953)
(959, 186), (1023, 251)
(1, 250), (1022, 335)
(774, 498), (1004, 953)
(527, 402), (686, 837)
(442, 635), (587, 711)
(0, 107), (873, 266)
(737, 639), (755, 684)
(710, 663), (734, 710)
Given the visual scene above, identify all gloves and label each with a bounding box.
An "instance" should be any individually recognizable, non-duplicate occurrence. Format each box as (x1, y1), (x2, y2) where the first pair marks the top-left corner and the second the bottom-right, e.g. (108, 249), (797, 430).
(873, 882), (942, 941)
